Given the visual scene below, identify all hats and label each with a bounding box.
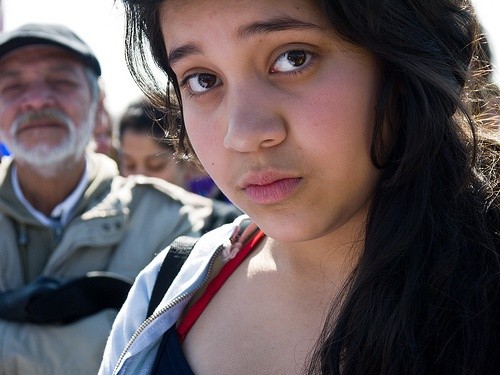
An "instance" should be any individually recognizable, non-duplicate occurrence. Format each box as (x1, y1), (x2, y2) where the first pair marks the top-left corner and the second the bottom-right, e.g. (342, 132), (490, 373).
(1, 24), (101, 76)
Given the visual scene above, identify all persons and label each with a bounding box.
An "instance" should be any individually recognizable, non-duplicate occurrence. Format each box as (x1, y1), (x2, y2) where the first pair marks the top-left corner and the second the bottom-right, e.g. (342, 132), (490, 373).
(101, 1), (500, 375)
(0, 21), (240, 375)
(98, 103), (121, 161)
(116, 98), (186, 188)
